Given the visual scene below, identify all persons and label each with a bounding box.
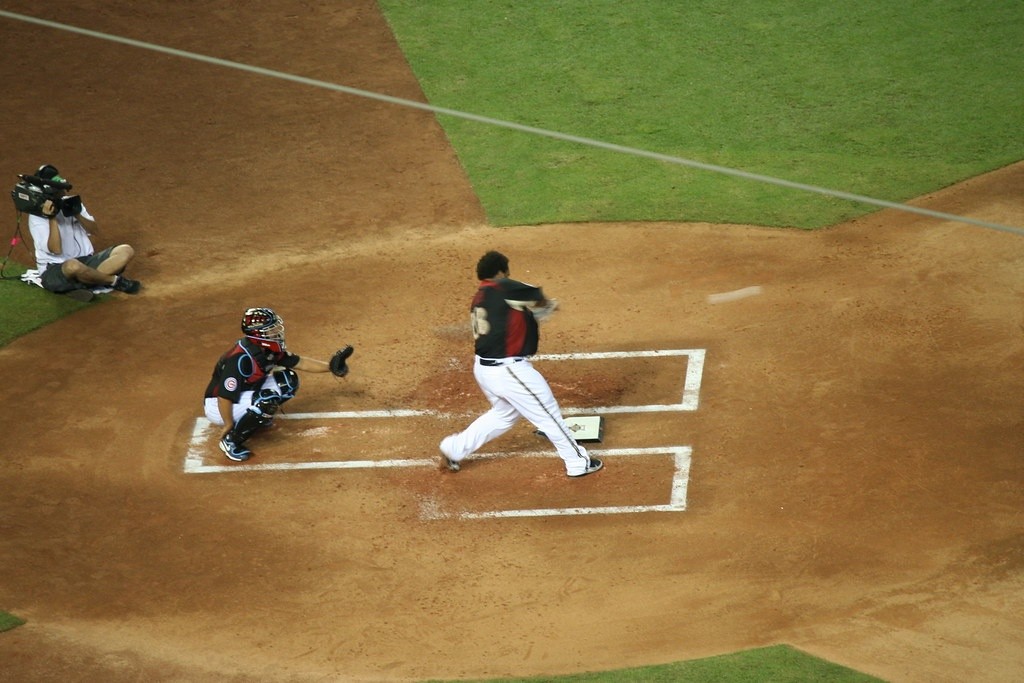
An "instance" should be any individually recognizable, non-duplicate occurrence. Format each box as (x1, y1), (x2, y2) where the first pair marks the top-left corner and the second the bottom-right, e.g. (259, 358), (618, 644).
(29, 163), (140, 302)
(202, 307), (355, 461)
(440, 250), (604, 476)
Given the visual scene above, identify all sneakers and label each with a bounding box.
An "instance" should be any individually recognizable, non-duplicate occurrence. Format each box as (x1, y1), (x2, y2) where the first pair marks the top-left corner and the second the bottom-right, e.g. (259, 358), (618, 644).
(110, 275), (140, 293)
(70, 290), (94, 301)
(219, 435), (250, 460)
(439, 444), (460, 472)
(567, 457), (603, 475)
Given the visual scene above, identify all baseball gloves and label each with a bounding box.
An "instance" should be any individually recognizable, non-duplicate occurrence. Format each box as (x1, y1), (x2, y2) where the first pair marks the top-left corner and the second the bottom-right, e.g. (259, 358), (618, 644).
(328, 344), (354, 377)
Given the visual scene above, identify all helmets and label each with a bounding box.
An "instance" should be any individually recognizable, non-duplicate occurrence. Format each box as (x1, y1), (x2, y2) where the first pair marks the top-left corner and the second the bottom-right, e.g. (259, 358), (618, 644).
(239, 306), (286, 352)
(33, 164), (58, 179)
